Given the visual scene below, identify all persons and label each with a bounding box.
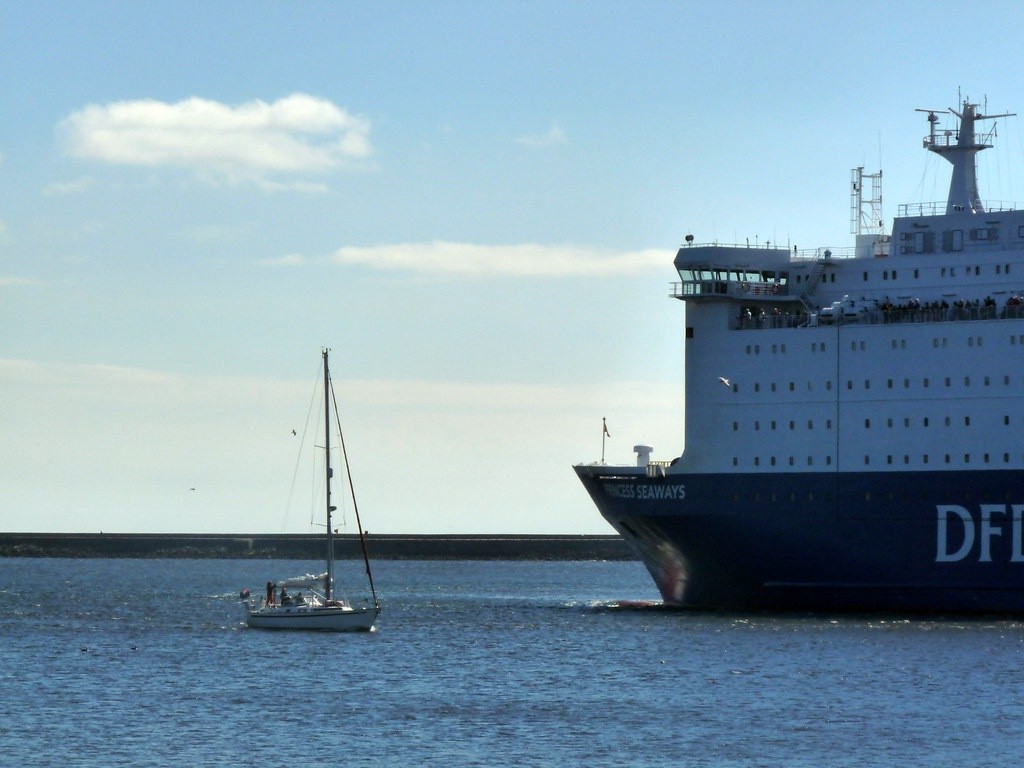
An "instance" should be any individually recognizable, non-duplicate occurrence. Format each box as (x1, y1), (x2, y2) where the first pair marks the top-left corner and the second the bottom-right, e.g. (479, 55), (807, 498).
(280, 588), (291, 601)
(881, 297), (996, 323)
(745, 306), (807, 328)
(1000, 296), (1024, 319)
(266, 581), (273, 607)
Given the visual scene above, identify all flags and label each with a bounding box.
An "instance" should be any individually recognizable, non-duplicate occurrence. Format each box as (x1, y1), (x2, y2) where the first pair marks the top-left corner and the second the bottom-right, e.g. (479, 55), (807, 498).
(605, 421), (610, 438)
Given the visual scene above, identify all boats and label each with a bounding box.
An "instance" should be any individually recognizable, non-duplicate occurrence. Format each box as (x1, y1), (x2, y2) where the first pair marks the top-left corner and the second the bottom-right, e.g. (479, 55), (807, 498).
(573, 85), (1023, 616)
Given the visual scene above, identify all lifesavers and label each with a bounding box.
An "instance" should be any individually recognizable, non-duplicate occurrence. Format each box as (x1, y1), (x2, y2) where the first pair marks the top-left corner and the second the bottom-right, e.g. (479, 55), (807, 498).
(769, 284), (783, 297)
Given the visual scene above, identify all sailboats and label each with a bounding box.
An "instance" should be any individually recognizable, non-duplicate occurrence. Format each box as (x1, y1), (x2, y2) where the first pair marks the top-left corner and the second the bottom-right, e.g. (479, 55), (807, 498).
(239, 349), (380, 627)
